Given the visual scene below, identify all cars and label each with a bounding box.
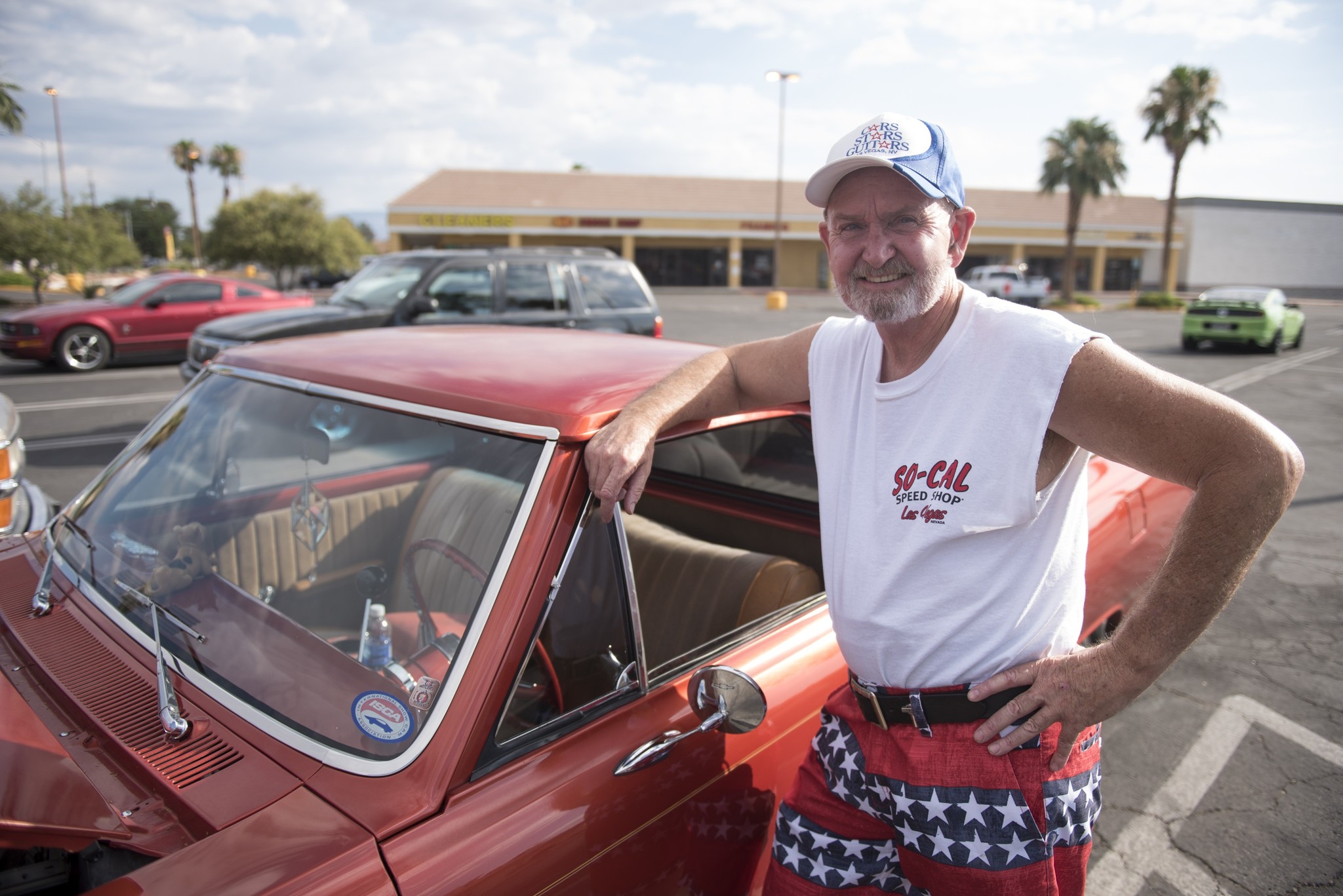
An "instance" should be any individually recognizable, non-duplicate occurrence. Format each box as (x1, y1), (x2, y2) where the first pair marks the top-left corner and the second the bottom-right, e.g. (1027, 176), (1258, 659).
(1181, 286), (1303, 358)
(1, 320), (1199, 896)
(0, 271), (320, 376)
(295, 266), (352, 292)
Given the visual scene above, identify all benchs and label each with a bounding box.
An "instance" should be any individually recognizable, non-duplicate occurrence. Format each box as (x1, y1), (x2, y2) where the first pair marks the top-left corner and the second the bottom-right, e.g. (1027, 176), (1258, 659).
(390, 463), (821, 676)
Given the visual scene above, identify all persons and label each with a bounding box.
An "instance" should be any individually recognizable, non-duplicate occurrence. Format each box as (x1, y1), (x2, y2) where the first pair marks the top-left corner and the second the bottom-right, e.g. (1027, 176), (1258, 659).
(585, 118), (1304, 896)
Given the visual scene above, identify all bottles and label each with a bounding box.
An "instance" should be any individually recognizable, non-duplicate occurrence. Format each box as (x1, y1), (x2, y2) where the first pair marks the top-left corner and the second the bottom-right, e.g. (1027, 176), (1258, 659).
(357, 604), (393, 671)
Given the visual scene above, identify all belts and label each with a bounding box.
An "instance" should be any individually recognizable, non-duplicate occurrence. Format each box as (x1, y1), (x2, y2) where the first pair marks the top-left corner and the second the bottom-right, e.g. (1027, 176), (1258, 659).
(841, 664), (1036, 732)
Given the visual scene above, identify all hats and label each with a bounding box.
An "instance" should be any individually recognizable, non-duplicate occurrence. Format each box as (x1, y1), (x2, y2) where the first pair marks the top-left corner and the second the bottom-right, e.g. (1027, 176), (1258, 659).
(804, 118), (966, 219)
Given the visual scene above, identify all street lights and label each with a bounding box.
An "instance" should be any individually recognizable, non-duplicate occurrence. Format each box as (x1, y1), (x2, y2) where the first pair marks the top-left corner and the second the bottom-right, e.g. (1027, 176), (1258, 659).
(44, 85), (72, 218)
(773, 69), (804, 291)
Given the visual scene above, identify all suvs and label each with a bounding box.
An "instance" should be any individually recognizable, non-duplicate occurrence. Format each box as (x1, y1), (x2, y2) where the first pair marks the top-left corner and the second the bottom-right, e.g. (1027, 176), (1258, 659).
(967, 259), (1053, 310)
(176, 246), (663, 448)
